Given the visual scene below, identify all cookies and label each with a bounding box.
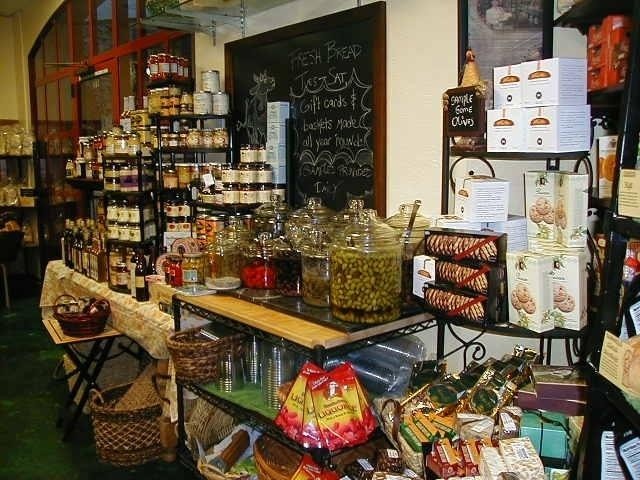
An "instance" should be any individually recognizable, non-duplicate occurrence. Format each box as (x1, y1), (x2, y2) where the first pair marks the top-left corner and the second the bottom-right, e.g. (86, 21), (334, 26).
(511, 284), (575, 314)
(529, 197), (567, 229)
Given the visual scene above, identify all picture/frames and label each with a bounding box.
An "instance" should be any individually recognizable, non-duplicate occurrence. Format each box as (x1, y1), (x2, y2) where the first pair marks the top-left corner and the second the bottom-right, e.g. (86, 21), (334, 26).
(456, 0), (557, 88)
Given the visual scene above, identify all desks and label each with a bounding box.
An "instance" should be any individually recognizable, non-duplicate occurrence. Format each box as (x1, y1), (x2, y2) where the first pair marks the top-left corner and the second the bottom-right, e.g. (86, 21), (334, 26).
(37, 257), (215, 477)
(39, 317), (125, 443)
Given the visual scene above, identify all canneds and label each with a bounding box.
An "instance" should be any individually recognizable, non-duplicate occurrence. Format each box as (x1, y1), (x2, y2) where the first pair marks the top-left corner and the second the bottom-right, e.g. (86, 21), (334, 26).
(77, 53), (285, 289)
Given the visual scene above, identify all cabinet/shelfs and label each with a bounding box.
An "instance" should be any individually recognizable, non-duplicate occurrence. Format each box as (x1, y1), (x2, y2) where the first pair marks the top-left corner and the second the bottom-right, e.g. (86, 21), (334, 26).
(433, 108), (596, 367)
(46, 148), (85, 254)
(101, 147), (159, 294)
(150, 113), (242, 276)
(169, 289), (451, 479)
(553, 0), (640, 479)
(0, 140), (47, 297)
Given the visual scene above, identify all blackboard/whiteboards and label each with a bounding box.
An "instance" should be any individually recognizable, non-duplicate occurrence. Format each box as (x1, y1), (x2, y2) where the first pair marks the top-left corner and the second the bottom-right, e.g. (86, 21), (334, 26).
(446, 85), (486, 136)
(224, 1), (386, 219)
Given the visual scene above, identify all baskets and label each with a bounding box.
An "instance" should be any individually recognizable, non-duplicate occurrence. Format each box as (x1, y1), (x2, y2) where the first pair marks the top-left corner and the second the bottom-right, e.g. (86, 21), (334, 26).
(168, 321), (251, 384)
(62, 336), (155, 414)
(89, 379), (167, 465)
(54, 294), (109, 335)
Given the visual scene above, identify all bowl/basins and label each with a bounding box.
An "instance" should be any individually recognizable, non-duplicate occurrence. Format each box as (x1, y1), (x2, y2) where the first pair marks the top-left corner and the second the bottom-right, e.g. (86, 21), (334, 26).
(217, 335), (296, 412)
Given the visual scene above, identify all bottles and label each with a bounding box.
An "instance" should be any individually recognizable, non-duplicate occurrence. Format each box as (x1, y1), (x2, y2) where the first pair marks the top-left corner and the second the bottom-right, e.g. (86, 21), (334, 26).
(105, 108), (155, 298)
(59, 207), (103, 283)
(159, 161), (221, 188)
(163, 192), (428, 323)
(65, 132), (104, 179)
(222, 142), (273, 204)
(148, 87), (193, 116)
(0, 125), (33, 155)
(147, 54), (190, 80)
(159, 126), (228, 148)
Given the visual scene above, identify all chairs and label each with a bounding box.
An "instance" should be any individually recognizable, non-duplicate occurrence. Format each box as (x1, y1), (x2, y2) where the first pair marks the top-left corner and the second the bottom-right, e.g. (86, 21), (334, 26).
(0, 229), (27, 312)
